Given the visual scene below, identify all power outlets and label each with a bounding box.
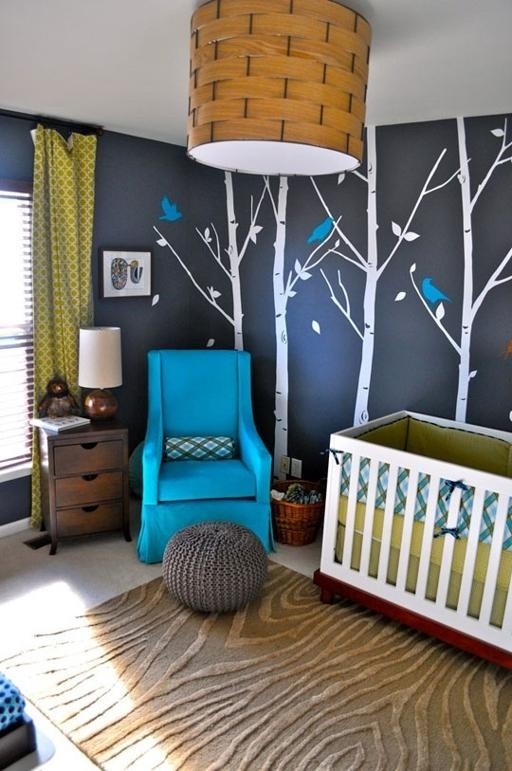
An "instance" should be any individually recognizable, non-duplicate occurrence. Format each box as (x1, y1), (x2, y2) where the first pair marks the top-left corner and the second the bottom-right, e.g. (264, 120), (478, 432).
(280, 456), (290, 474)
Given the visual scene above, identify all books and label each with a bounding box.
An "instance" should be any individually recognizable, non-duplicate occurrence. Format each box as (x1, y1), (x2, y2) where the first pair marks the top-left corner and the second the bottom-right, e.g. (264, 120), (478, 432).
(29, 416), (90, 432)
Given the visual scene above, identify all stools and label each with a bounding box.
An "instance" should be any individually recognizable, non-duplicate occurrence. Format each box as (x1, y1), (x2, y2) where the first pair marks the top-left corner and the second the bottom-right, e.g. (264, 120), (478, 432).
(162, 520), (269, 615)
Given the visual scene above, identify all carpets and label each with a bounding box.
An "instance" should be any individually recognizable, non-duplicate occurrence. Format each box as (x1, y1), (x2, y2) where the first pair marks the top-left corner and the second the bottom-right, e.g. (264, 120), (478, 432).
(0, 555), (511, 771)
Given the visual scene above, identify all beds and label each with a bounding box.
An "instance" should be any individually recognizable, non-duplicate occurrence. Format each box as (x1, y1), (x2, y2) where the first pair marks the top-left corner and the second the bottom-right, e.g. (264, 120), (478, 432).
(314, 410), (512, 671)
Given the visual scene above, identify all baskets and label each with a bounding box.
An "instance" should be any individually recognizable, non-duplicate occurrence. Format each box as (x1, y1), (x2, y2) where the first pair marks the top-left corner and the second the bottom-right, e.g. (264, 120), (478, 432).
(272, 479), (324, 546)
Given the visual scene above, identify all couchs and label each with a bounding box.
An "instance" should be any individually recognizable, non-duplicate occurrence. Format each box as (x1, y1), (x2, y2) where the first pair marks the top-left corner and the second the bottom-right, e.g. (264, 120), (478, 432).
(137, 349), (276, 564)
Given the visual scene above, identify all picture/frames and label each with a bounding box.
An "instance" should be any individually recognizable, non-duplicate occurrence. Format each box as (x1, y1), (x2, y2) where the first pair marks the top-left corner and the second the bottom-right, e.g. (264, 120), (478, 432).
(98, 247), (153, 299)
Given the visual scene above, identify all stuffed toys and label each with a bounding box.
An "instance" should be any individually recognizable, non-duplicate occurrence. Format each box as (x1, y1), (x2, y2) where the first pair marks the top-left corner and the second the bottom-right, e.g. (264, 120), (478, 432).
(38, 379), (79, 419)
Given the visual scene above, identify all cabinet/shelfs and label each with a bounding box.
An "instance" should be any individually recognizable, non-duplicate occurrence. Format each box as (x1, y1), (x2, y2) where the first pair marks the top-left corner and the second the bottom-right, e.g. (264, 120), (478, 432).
(39, 422), (132, 555)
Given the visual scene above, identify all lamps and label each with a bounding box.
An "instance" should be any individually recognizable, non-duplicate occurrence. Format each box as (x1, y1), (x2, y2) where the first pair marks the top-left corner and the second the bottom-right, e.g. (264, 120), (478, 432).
(185, 0), (373, 177)
(78, 326), (123, 425)
